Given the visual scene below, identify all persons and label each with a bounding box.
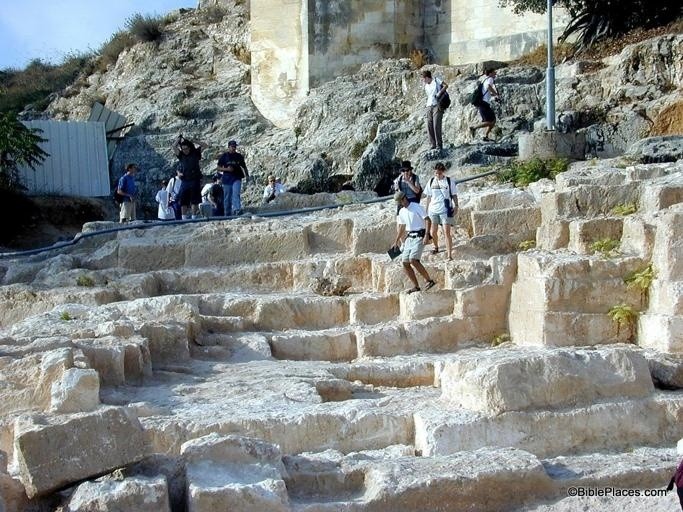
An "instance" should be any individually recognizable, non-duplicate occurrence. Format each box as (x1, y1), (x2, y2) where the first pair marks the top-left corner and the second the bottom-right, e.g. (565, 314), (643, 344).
(672, 461), (682, 511)
(260, 174), (282, 202)
(393, 161), (421, 215)
(465, 67), (498, 142)
(152, 134), (250, 219)
(421, 162), (458, 260)
(420, 67), (447, 153)
(115, 163), (139, 223)
(393, 189), (435, 292)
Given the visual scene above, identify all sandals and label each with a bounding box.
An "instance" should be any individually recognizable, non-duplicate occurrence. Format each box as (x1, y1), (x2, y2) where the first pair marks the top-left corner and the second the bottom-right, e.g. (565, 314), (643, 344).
(406, 286), (421, 294)
(431, 247), (439, 255)
(423, 280), (436, 291)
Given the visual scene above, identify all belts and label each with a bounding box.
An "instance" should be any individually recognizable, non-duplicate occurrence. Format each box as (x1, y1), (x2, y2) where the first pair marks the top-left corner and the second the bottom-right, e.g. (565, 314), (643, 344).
(406, 233), (420, 238)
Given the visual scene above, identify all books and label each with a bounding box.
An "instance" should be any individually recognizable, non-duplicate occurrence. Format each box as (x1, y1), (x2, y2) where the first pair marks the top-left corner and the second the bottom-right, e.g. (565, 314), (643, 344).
(386, 245), (401, 258)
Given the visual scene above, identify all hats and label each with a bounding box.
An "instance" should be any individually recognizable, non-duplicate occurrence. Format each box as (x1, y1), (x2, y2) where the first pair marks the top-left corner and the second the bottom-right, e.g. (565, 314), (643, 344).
(394, 191), (405, 201)
(399, 160), (413, 171)
(227, 140), (239, 147)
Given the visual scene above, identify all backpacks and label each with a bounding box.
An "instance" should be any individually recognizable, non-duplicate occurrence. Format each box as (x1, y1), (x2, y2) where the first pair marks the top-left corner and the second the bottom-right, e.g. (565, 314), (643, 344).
(178, 135), (195, 152)
(434, 77), (451, 112)
(112, 175), (128, 206)
(470, 76), (491, 107)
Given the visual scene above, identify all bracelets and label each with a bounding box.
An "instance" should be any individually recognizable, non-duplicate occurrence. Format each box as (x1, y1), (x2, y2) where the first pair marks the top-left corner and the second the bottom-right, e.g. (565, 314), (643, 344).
(455, 204), (458, 208)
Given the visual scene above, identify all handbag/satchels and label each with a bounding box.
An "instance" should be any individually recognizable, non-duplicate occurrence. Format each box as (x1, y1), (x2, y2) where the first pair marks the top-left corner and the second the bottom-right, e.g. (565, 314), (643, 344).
(409, 230), (433, 240)
(447, 206), (455, 218)
(167, 190), (178, 205)
